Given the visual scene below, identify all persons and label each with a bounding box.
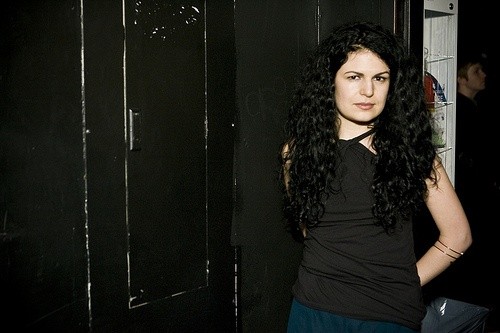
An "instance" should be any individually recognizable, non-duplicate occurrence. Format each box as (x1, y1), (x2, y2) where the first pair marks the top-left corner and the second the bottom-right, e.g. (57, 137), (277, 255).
(282, 22), (472, 333)
(451, 55), (493, 214)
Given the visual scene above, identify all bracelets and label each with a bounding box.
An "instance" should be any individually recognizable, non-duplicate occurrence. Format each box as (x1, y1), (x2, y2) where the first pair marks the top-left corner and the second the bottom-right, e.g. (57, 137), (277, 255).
(434, 240), (461, 260)
